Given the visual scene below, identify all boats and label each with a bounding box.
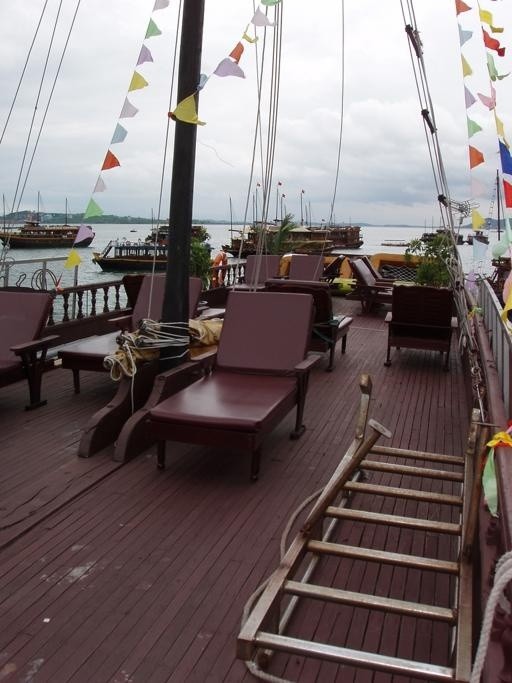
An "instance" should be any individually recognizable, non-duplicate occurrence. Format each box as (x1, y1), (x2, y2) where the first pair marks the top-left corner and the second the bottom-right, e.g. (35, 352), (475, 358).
(91, 238), (214, 272)
(221, 223), (334, 257)
(419, 228), (489, 245)
(145, 225), (206, 248)
(0, 219), (95, 248)
(229, 220), (363, 249)
(380, 239), (410, 247)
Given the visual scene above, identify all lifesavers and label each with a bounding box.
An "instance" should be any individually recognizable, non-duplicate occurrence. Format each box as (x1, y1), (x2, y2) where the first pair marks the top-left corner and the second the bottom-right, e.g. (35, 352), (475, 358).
(211, 251), (228, 288)
